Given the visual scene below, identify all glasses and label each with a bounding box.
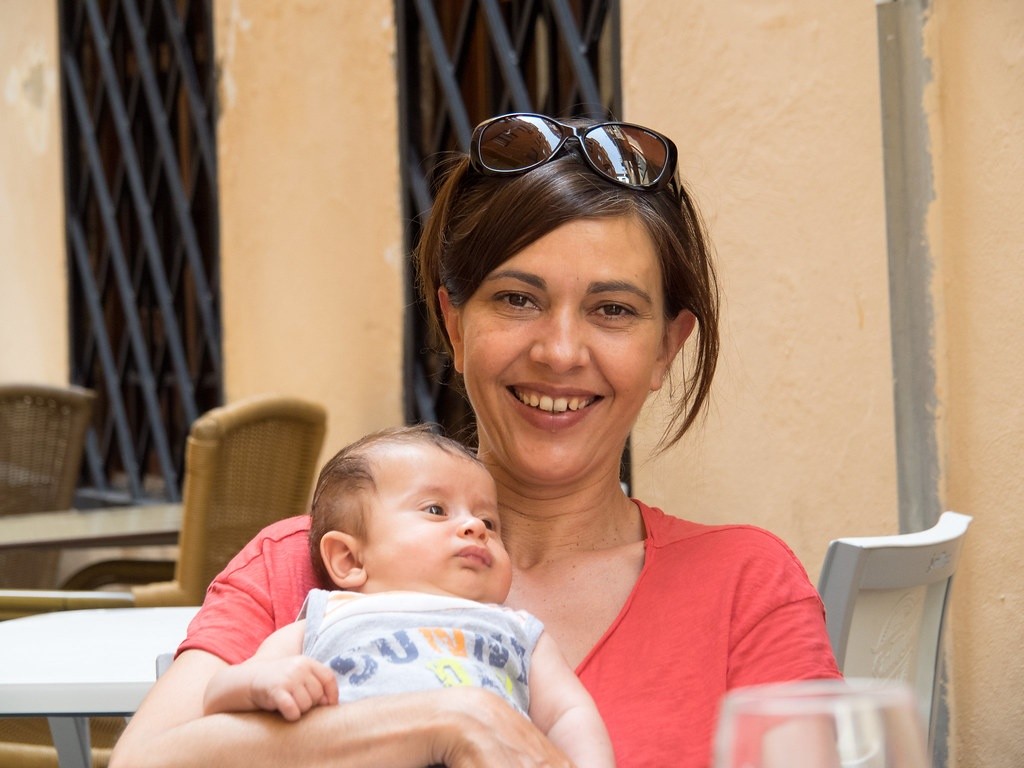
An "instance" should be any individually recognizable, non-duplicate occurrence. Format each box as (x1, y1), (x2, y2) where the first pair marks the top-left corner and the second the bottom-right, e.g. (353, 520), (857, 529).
(464, 112), (681, 202)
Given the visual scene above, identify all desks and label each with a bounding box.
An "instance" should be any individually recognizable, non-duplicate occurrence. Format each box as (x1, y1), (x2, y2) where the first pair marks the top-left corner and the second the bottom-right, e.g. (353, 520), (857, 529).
(0, 604), (201, 768)
(0, 498), (184, 553)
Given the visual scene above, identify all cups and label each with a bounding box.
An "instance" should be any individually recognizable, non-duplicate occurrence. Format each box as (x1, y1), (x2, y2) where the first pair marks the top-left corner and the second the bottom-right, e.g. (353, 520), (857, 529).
(711, 678), (931, 768)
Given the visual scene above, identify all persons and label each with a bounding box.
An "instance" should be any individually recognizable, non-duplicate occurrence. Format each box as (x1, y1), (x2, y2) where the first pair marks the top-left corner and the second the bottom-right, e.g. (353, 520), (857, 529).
(200, 425), (615, 768)
(109, 114), (843, 768)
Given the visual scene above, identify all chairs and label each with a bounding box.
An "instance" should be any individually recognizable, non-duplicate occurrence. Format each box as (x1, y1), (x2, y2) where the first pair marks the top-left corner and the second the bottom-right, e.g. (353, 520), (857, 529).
(818, 507), (976, 765)
(0, 373), (99, 593)
(0, 391), (337, 612)
